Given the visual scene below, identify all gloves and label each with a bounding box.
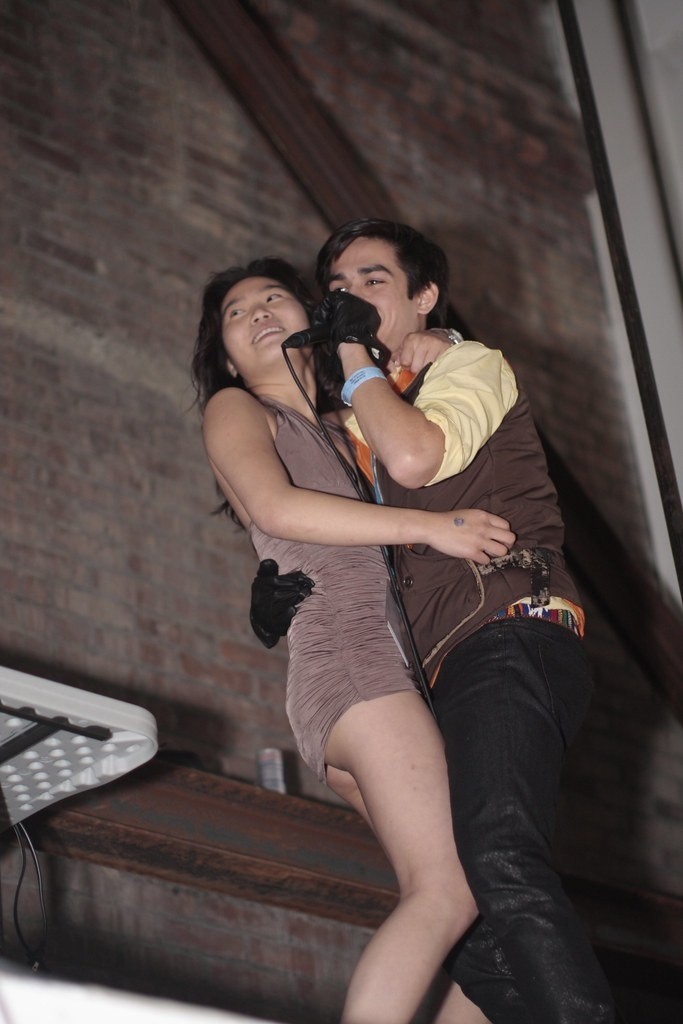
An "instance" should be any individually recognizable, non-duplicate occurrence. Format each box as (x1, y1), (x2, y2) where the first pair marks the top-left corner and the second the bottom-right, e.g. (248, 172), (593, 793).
(311, 290), (380, 354)
(250, 558), (315, 649)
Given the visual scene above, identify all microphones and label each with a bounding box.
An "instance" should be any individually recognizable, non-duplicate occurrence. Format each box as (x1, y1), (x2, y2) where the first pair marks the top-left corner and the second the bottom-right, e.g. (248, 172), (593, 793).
(282, 325), (331, 349)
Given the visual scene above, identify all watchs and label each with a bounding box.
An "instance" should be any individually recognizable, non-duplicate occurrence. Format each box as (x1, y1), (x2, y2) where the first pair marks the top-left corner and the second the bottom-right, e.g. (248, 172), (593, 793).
(430, 327), (465, 344)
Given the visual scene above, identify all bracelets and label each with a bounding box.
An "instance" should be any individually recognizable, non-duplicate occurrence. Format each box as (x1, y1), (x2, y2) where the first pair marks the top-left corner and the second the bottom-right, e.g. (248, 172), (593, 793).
(341, 366), (387, 406)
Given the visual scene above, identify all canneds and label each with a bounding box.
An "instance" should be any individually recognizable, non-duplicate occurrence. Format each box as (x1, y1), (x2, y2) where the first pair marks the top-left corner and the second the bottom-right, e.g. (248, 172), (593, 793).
(253, 748), (286, 794)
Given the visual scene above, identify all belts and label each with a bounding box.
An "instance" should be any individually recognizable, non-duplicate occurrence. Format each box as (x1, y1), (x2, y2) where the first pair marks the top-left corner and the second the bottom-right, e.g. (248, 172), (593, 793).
(483, 603), (581, 639)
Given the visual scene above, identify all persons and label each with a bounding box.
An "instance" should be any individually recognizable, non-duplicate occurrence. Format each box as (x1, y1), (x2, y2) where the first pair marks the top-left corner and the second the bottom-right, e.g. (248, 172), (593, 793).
(248, 220), (625, 1024)
(191, 257), (514, 1024)
